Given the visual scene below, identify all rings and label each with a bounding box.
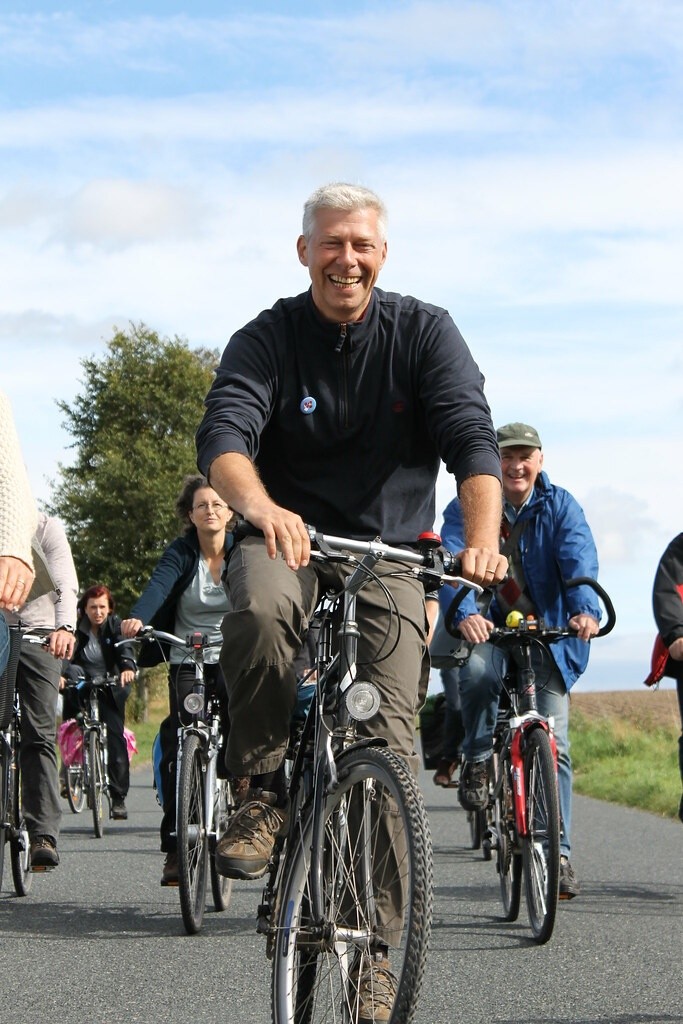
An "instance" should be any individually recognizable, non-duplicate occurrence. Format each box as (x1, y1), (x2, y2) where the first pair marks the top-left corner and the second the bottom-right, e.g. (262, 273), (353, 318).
(18, 580), (25, 585)
(486, 570), (495, 574)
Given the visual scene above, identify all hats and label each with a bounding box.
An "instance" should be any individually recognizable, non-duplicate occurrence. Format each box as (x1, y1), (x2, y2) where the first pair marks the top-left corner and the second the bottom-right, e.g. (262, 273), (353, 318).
(496, 423), (542, 449)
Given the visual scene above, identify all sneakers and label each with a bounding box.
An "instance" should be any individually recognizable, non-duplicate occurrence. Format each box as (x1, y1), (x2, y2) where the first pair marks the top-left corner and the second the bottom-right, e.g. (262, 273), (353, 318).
(347, 953), (398, 1024)
(30, 835), (60, 866)
(560, 856), (579, 899)
(459, 761), (489, 812)
(161, 853), (182, 886)
(60, 783), (74, 798)
(112, 802), (127, 819)
(433, 764), (455, 788)
(215, 788), (292, 880)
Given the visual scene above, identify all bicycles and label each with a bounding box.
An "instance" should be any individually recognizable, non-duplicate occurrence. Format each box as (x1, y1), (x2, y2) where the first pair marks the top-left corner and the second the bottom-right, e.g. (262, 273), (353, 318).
(60, 672), (137, 838)
(233, 520), (510, 1024)
(444, 575), (617, 944)
(114, 626), (235, 936)
(0, 620), (56, 897)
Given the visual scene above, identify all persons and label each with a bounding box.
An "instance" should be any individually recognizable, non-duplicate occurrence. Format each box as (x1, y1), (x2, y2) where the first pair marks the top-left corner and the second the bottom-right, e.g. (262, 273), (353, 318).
(1, 513), (78, 867)
(438, 422), (603, 894)
(60, 584), (137, 820)
(196, 184), (509, 1024)
(429, 620), (463, 785)
(641, 534), (683, 819)
(1, 401), (35, 673)
(119, 478), (233, 886)
